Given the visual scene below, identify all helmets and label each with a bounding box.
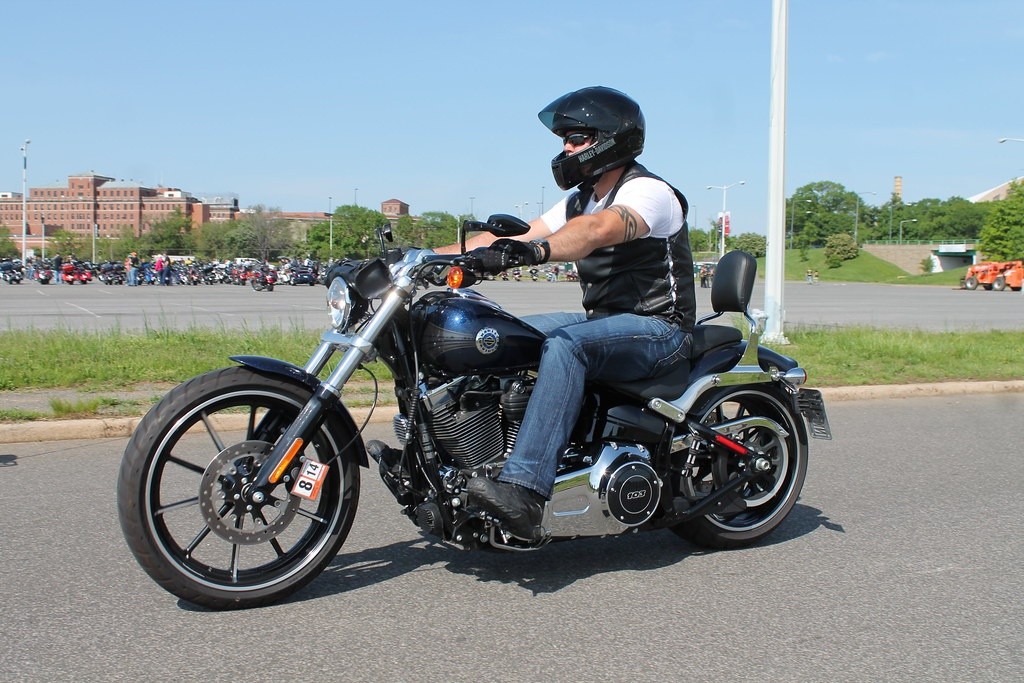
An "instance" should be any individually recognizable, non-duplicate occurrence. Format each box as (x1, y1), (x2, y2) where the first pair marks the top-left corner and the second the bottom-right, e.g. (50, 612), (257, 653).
(538, 86), (647, 192)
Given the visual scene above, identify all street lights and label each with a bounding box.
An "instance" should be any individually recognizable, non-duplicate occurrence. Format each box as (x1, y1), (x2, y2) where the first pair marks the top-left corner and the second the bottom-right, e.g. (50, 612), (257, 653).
(542, 186), (545, 216)
(325, 213), (333, 250)
(790, 200), (812, 252)
(889, 204), (912, 243)
(515, 202), (529, 220)
(854, 192), (879, 242)
(19, 139), (31, 269)
(707, 181), (746, 257)
(469, 197), (475, 215)
(354, 188), (359, 202)
(536, 202), (542, 218)
(691, 205), (697, 265)
(900, 219), (918, 245)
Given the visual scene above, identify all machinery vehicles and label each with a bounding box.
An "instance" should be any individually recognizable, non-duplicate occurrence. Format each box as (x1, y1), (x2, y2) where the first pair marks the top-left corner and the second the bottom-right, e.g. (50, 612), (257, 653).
(960, 261), (1024, 292)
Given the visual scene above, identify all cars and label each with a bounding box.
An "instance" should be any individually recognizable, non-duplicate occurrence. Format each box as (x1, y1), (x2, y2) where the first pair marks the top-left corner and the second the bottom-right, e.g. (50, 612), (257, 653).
(477, 272), (496, 281)
(234, 258), (275, 270)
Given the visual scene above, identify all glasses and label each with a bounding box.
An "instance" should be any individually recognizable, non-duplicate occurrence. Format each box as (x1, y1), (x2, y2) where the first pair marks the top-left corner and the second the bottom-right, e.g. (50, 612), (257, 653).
(563, 133), (593, 145)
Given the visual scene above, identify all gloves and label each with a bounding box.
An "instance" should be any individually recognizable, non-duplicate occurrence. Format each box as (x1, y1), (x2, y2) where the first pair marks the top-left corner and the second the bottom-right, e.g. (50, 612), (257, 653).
(482, 237), (540, 276)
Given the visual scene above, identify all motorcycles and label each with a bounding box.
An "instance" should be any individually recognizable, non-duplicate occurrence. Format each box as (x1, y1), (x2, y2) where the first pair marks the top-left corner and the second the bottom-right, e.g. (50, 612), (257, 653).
(500, 268), (579, 282)
(0, 258), (329, 292)
(116, 218), (833, 612)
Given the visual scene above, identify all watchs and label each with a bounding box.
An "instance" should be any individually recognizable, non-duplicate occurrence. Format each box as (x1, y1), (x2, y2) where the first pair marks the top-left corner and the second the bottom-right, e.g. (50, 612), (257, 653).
(529, 238), (550, 264)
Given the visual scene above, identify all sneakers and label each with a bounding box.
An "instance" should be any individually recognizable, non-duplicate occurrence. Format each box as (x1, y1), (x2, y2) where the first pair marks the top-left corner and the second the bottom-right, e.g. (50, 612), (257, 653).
(466, 476), (544, 541)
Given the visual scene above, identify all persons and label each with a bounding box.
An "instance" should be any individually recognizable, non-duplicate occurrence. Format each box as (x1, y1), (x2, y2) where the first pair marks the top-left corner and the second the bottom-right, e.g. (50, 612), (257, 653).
(550, 263), (560, 282)
(179, 257), (192, 266)
(211, 257), (231, 266)
(125, 250), (171, 286)
(325, 86), (696, 541)
(2, 253), (76, 285)
(700, 264), (714, 288)
(279, 257), (291, 269)
(806, 268), (819, 285)
